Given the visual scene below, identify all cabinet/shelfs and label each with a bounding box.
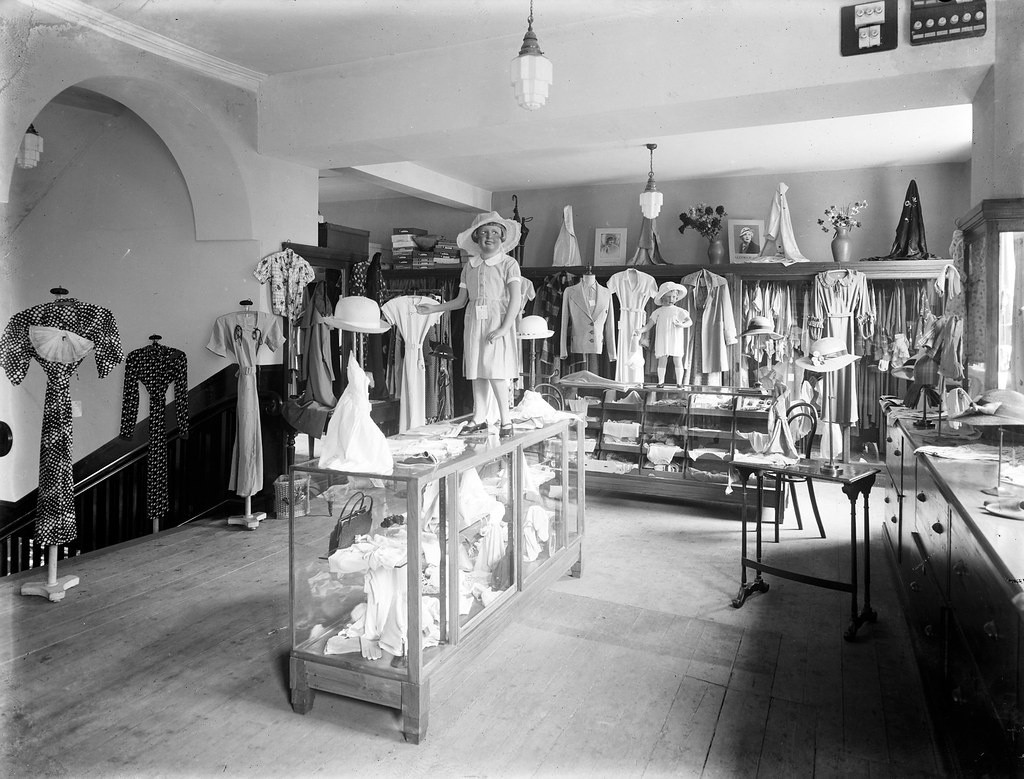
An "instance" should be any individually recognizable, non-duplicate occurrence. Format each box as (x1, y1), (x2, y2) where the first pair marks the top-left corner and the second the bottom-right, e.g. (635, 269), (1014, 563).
(880, 400), (1023, 777)
(547, 378), (793, 521)
(290, 411), (588, 741)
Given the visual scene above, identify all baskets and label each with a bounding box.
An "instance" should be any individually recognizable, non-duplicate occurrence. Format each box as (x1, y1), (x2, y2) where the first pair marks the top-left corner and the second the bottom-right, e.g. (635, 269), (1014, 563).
(273, 474), (311, 519)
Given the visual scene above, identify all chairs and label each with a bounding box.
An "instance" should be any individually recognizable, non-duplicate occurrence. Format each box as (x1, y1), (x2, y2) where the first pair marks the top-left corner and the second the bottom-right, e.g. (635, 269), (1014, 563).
(742, 402), (826, 542)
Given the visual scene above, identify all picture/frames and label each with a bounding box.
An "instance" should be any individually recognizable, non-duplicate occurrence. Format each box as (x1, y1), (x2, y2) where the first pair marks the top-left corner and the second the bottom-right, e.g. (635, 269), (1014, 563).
(594, 228), (628, 266)
(728, 219), (764, 263)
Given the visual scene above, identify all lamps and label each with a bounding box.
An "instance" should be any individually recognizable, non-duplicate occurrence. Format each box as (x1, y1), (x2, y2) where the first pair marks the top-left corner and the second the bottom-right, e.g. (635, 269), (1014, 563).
(511, 0), (552, 109)
(639, 142), (663, 218)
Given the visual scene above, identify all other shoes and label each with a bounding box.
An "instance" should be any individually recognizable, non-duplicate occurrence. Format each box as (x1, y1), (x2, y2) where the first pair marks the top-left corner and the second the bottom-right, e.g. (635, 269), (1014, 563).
(499, 421), (514, 437)
(459, 419), (487, 436)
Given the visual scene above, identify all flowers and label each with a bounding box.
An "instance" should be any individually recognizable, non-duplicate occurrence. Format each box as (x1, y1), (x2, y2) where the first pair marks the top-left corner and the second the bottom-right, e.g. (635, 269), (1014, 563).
(818, 200), (868, 233)
(678, 202), (727, 240)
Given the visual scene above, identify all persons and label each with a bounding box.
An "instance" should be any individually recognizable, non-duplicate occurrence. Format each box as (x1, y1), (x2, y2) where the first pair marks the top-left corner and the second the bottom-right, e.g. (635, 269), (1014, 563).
(560, 274), (617, 362)
(633, 282), (692, 389)
(739, 227), (760, 254)
(416, 211), (522, 436)
(601, 236), (619, 258)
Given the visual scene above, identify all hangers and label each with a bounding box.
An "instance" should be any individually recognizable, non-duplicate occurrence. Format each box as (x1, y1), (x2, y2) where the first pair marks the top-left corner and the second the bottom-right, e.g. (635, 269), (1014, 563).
(827, 261), (849, 274)
(401, 287), (423, 301)
(146, 333), (173, 352)
(236, 297), (258, 315)
(284, 239), (294, 258)
(47, 284), (76, 306)
(554, 260), (710, 287)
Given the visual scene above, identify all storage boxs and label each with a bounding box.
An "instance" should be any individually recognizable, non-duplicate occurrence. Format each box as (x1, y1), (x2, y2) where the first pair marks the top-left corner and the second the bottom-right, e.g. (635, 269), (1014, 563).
(318, 222), (460, 270)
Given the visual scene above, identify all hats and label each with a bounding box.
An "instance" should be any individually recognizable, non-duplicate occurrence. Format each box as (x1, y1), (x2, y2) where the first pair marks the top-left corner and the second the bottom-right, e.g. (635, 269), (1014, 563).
(320, 296), (392, 333)
(794, 336), (862, 372)
(654, 282), (687, 305)
(457, 210), (521, 255)
(891, 353), (925, 380)
(516, 315), (555, 339)
(738, 226), (753, 237)
(429, 344), (458, 360)
(951, 388), (1024, 425)
(735, 315), (784, 339)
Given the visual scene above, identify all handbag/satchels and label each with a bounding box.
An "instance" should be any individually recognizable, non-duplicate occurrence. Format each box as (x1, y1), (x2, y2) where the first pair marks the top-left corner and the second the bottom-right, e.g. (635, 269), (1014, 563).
(330, 491), (373, 553)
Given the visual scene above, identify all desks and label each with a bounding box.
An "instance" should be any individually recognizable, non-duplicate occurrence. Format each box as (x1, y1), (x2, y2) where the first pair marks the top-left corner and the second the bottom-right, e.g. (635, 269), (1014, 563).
(730, 451), (880, 641)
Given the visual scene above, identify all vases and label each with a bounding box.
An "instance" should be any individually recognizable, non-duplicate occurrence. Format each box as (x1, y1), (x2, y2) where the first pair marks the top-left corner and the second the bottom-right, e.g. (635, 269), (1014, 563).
(709, 239), (726, 264)
(832, 227), (853, 261)
